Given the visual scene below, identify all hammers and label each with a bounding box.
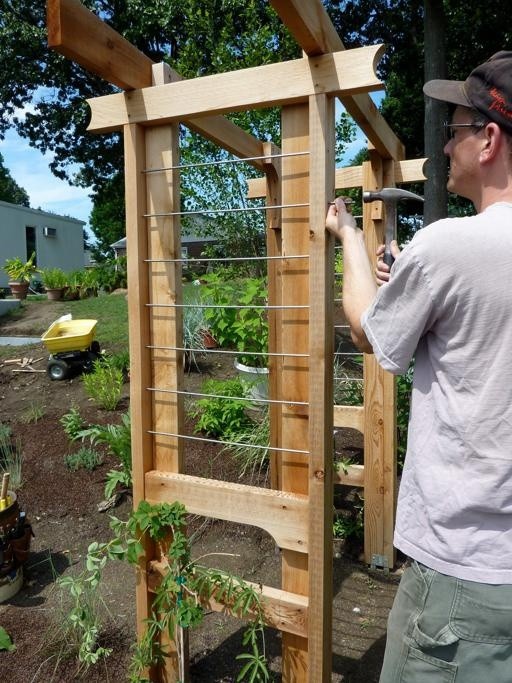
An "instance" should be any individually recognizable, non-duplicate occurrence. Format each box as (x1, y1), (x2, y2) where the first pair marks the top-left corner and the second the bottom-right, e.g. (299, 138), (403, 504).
(363, 188), (426, 281)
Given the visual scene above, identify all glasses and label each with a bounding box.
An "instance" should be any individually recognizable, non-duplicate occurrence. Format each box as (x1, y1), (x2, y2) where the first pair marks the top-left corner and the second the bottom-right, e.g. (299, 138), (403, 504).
(440, 117), (486, 141)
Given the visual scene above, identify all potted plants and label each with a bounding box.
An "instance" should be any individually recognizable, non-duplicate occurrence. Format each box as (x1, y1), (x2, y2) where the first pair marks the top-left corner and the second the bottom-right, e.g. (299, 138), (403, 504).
(1, 251), (96, 301)
(195, 271), (269, 408)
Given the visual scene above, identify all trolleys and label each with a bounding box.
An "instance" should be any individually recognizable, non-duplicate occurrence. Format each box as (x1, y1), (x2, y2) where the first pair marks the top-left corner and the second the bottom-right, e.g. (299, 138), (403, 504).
(41, 317), (105, 382)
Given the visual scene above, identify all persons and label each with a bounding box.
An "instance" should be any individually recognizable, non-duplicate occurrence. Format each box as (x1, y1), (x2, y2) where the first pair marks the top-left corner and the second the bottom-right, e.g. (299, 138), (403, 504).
(325, 49), (511, 682)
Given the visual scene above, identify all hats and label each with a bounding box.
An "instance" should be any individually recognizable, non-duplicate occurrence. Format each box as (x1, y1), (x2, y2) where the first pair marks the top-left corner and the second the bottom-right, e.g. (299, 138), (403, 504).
(418, 45), (512, 135)
